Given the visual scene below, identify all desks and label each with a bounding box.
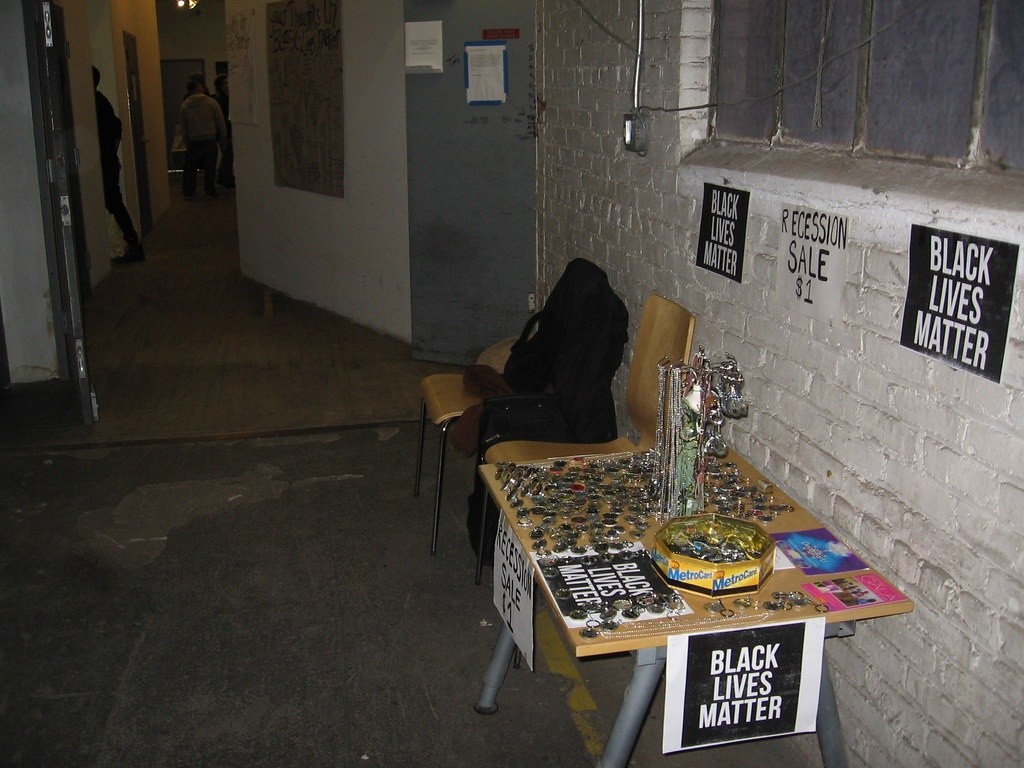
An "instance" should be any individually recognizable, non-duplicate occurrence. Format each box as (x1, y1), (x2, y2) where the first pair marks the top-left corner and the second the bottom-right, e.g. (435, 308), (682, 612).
(477, 441), (918, 767)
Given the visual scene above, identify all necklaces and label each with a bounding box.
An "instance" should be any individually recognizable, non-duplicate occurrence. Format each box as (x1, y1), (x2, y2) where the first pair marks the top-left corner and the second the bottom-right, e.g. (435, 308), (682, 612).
(652, 366), (680, 525)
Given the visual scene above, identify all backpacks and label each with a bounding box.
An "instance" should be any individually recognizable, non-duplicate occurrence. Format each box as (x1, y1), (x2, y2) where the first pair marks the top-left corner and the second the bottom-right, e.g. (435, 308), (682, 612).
(506, 258), (629, 396)
(466, 398), (578, 567)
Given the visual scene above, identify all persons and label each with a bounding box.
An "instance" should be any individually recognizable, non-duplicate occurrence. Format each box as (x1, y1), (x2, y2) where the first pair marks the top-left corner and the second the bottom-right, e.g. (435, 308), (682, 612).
(92, 66), (145, 266)
(180, 71), (235, 200)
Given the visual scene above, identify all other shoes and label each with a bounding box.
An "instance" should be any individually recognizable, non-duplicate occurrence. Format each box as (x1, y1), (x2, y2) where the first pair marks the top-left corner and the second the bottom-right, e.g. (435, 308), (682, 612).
(218, 176), (231, 186)
(184, 194), (191, 201)
(111, 242), (146, 265)
(205, 193), (218, 200)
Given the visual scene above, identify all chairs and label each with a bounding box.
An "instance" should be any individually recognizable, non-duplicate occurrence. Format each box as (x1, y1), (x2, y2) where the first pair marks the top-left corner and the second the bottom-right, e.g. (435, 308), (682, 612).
(413, 258), (613, 555)
(468, 290), (696, 594)
